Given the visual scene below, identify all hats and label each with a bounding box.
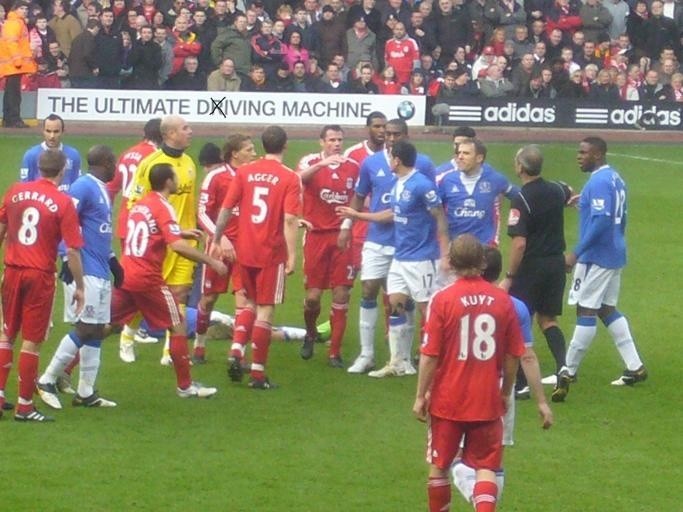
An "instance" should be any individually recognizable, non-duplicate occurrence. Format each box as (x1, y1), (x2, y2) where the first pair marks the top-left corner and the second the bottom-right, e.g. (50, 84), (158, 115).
(323, 5), (334, 13)
(12, 1), (28, 12)
(414, 68), (422, 72)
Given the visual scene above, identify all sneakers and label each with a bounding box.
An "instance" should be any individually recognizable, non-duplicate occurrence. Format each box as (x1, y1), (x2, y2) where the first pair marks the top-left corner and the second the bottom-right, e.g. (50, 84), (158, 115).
(56, 374), (75, 394)
(192, 339), (207, 365)
(14, 407), (55, 422)
(248, 376), (279, 390)
(161, 356), (193, 368)
(611, 365), (648, 386)
(300, 335), (315, 360)
(72, 393), (117, 407)
(317, 321), (331, 342)
(227, 355), (244, 382)
(326, 351), (343, 368)
(347, 354), (376, 374)
(3, 401), (14, 410)
(119, 330), (136, 362)
(551, 369), (569, 402)
(133, 327), (159, 343)
(541, 370), (577, 385)
(405, 364), (417, 375)
(35, 379), (63, 409)
(367, 361), (406, 378)
(176, 382), (217, 399)
(514, 385), (530, 400)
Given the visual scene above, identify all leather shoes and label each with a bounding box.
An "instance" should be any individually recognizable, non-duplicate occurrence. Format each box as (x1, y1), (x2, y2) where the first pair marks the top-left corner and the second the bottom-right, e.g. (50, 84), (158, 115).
(6, 121), (28, 127)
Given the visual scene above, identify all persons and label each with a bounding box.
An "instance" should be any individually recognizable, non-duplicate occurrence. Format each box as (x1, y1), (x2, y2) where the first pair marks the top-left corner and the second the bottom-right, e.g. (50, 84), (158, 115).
(173, 7), (194, 31)
(294, 123), (360, 369)
(136, 15), (148, 41)
(251, 0), (270, 22)
(275, 4), (295, 27)
(435, 126), (475, 190)
(286, 60), (315, 92)
(164, 0), (185, 28)
(419, 2), (432, 16)
(426, 0), (474, 55)
(613, 71), (627, 102)
(489, 28), (505, 56)
(428, 43), (443, 69)
(446, 58), (458, 71)
(545, 0), (580, 38)
(386, 24), (418, 91)
(344, 111), (393, 347)
(28, 56), (61, 91)
(150, 10), (170, 32)
(407, 11), (436, 53)
(541, 66), (556, 98)
(503, 40), (520, 78)
(20, 113), (82, 341)
(188, 9), (216, 49)
(56, 163), (216, 399)
(472, 45), (495, 88)
(572, 31), (585, 63)
(163, 55), (207, 90)
(466, 0), (499, 25)
(191, 133), (257, 372)
(497, 143), (571, 404)
(670, 73), (683, 102)
(127, 25), (161, 90)
(320, 63), (347, 93)
(453, 69), (469, 88)
(69, 19), (101, 90)
(476, 64), (514, 98)
(246, 10), (261, 36)
(511, 52), (535, 97)
(579, 0), (612, 42)
(650, 43), (679, 73)
(584, 64), (598, 87)
(401, 67), (432, 97)
(609, 47), (627, 67)
(43, 41), (70, 88)
(226, 0), (243, 24)
(658, 58), (674, 86)
(603, 0), (629, 40)
(540, 135), (649, 388)
(557, 70), (586, 99)
(637, 70), (671, 102)
(241, 63), (273, 92)
(449, 242), (553, 509)
(383, 15), (403, 48)
(588, 69), (619, 99)
(336, 117), (436, 375)
(622, 64), (640, 101)
(575, 40), (601, 69)
(345, 12), (377, 68)
(283, 6), (320, 53)
(207, 57), (241, 91)
(605, 64), (617, 87)
(330, 0), (347, 24)
(119, 31), (134, 76)
(320, 52), (349, 84)
(525, 71), (549, 98)
(134, 0), (157, 24)
(32, 144), (119, 411)
(0, 4), (6, 23)
(269, 63), (290, 91)
(110, 0), (128, 31)
(347, 0), (380, 33)
(414, 70), (456, 98)
(524, 0), (544, 22)
(210, 0), (230, 26)
(30, 14), (56, 58)
(438, 137), (522, 248)
(617, 55), (651, 84)
(421, 53), (443, 90)
(105, 115), (162, 258)
(379, 64), (401, 95)
(382, 0), (414, 35)
(118, 115), (195, 369)
(310, 4), (348, 70)
(350, 63), (378, 94)
(96, 8), (123, 89)
(305, 51), (324, 77)
(452, 46), (471, 75)
(209, 125), (303, 391)
(197, 0), (215, 15)
(171, 16), (202, 76)
(497, 0), (527, 39)
(153, 24), (174, 86)
(127, 10), (137, 39)
(544, 27), (565, 58)
(594, 33), (620, 69)
(46, 0), (82, 58)
(71, 0), (98, 23)
(87, 3), (102, 18)
(528, 20), (547, 45)
(334, 139), (450, 379)
(626, 0), (649, 49)
(609, 33), (634, 62)
(0, 148), (85, 424)
(249, 20), (287, 67)
(0, 1), (38, 132)
(140, 301), (333, 341)
(497, 55), (506, 72)
(532, 41), (548, 68)
(512, 24), (534, 59)
(641, 0), (680, 58)
(550, 46), (580, 79)
(271, 20), (289, 44)
(412, 233), (528, 512)
(282, 31), (308, 70)
(351, 54), (379, 83)
(304, 0), (321, 25)
(210, 13), (252, 73)
